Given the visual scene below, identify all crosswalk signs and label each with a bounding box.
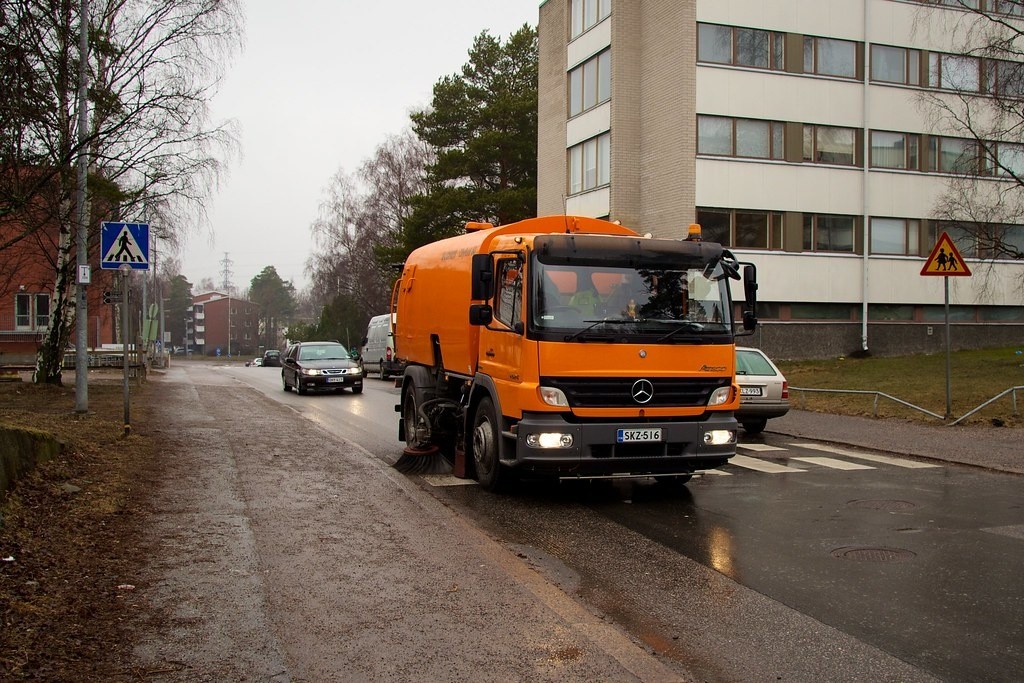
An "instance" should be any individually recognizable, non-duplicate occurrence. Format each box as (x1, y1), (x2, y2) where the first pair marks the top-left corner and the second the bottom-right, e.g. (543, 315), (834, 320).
(98, 222), (151, 271)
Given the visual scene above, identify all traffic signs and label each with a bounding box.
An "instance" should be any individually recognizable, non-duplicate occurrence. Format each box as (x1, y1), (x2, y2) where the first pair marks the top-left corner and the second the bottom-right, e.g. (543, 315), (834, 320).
(104, 296), (130, 304)
(104, 290), (130, 297)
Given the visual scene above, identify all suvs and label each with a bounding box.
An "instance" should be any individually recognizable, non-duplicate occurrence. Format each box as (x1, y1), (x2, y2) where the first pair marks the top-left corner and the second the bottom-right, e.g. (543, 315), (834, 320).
(280, 338), (363, 396)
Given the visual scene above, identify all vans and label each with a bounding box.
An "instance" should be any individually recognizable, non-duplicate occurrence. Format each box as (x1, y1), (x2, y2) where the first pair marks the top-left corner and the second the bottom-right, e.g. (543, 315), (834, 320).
(360, 313), (406, 381)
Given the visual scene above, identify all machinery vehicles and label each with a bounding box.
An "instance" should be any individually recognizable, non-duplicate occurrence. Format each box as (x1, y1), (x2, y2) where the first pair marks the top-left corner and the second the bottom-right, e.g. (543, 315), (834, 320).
(385, 214), (760, 495)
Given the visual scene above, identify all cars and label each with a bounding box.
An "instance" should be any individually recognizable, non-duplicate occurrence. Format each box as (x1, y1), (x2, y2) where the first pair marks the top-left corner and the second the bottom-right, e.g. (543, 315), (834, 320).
(261, 349), (283, 367)
(253, 357), (262, 367)
(735, 345), (792, 436)
(158, 347), (195, 356)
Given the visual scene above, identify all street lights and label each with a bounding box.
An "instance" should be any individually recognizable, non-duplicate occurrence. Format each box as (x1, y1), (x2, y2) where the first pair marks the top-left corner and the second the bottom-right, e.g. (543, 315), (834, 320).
(153, 234), (169, 360)
(141, 170), (168, 365)
